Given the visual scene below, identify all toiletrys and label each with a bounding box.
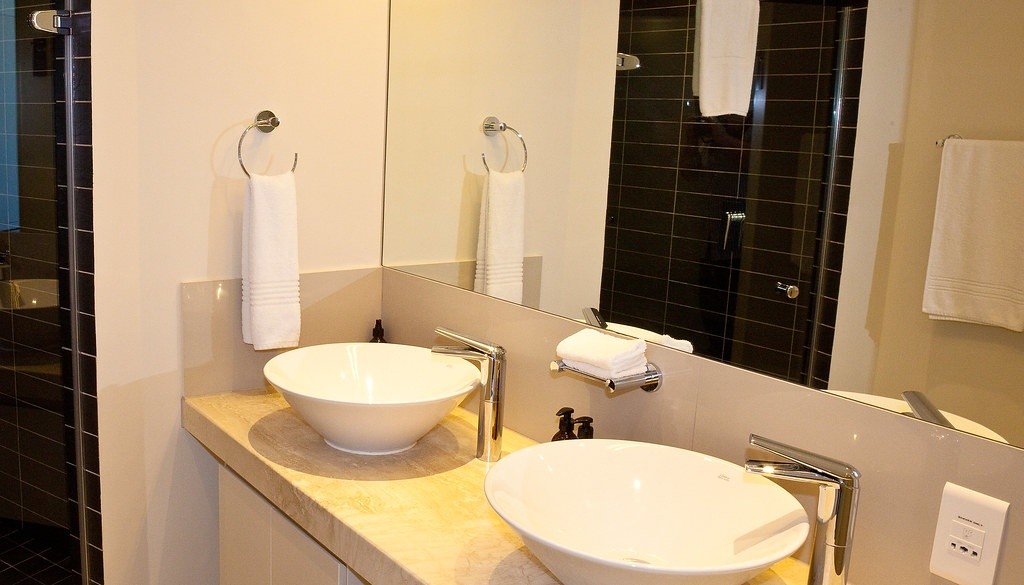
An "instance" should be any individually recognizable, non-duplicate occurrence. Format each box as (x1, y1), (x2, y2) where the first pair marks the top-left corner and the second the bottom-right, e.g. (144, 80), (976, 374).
(368, 317), (387, 343)
(549, 404), (596, 441)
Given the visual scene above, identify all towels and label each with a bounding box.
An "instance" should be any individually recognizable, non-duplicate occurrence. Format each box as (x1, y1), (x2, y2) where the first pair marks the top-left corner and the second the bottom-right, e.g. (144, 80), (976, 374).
(240, 168), (303, 355)
(556, 328), (648, 381)
(693, 0), (761, 118)
(920, 136), (1020, 332)
(471, 168), (525, 305)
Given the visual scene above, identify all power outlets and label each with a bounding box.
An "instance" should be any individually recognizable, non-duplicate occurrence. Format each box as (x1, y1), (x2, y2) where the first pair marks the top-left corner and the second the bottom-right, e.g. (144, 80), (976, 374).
(929, 481), (1011, 585)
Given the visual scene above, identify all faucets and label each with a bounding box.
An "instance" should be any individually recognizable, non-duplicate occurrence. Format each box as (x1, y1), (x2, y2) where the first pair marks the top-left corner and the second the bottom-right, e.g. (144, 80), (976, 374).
(583, 306), (609, 331)
(428, 324), (508, 462)
(893, 390), (955, 430)
(742, 431), (863, 585)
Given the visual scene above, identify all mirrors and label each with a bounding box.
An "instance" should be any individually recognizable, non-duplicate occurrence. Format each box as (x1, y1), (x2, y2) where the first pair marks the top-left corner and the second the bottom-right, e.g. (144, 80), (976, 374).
(382, 0), (1024, 450)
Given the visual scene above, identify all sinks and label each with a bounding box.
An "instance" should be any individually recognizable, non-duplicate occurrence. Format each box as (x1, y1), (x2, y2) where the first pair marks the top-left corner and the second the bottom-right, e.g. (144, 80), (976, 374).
(482, 436), (811, 585)
(261, 340), (481, 458)
(574, 318), (695, 355)
(825, 388), (1010, 445)
